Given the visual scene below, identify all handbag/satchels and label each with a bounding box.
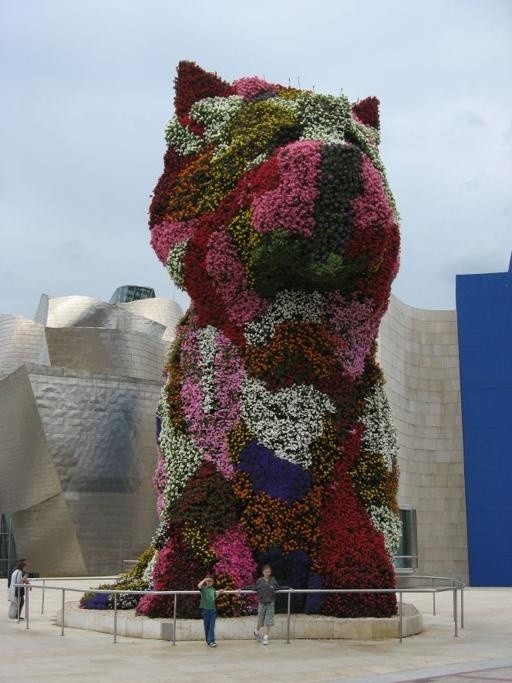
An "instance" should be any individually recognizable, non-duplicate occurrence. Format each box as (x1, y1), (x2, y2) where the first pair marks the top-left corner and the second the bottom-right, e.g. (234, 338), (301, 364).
(15, 587), (25, 597)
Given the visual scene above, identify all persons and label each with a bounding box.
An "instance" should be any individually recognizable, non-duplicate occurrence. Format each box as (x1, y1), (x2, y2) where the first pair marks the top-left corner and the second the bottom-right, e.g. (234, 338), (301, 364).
(6, 563), (28, 621)
(196, 574), (225, 647)
(8, 557), (39, 619)
(234, 563), (293, 645)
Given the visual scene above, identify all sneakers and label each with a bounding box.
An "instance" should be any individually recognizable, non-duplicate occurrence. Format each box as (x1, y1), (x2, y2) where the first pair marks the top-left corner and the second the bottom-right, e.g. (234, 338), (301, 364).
(8, 616), (24, 622)
(253, 631), (269, 645)
(209, 643), (217, 647)
